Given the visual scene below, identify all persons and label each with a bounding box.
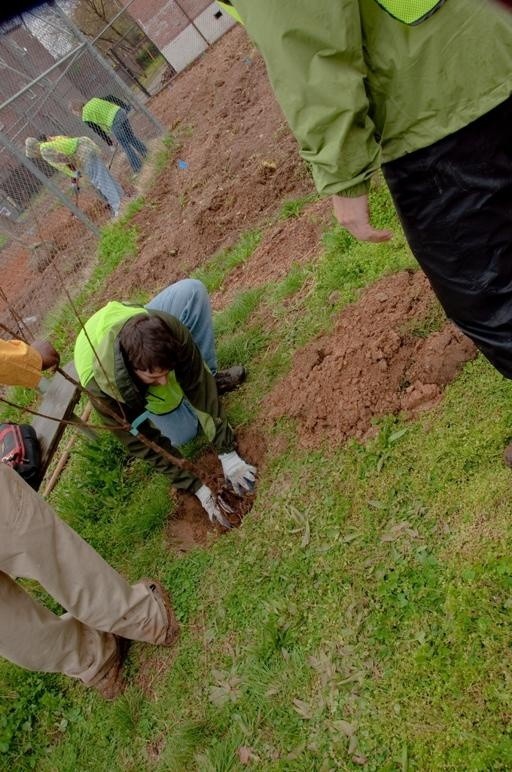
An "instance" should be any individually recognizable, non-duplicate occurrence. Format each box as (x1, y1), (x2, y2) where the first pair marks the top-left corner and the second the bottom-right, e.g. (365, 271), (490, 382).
(0, 339), (180, 704)
(37, 134), (110, 207)
(232, 0), (511, 382)
(73, 276), (259, 525)
(68, 94), (148, 180)
(25, 137), (130, 220)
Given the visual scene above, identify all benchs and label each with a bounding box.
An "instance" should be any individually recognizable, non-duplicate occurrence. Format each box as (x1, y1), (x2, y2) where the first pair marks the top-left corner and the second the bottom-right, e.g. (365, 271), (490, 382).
(21, 353), (103, 517)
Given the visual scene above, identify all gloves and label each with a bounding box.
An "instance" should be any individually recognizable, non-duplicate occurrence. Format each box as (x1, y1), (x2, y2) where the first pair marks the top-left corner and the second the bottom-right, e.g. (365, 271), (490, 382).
(218, 450), (257, 497)
(195, 486), (235, 529)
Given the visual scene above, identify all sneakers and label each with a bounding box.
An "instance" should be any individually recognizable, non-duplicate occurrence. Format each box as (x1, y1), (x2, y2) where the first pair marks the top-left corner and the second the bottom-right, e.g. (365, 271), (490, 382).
(94, 633), (128, 701)
(215, 366), (245, 395)
(137, 577), (179, 646)
